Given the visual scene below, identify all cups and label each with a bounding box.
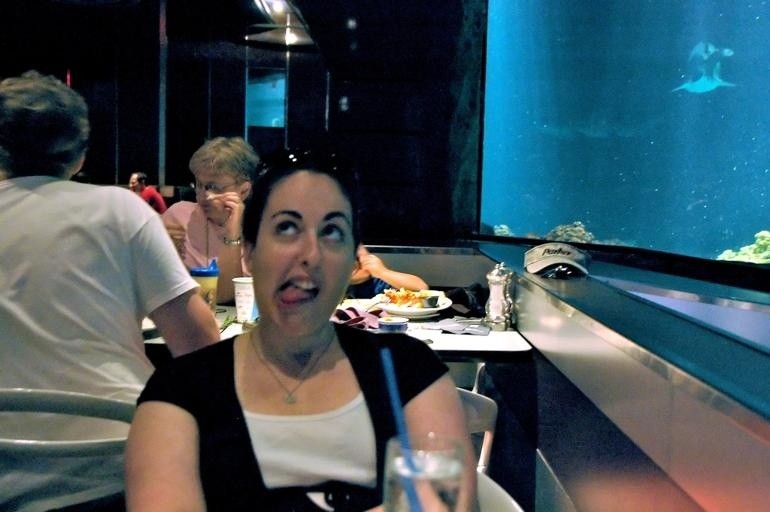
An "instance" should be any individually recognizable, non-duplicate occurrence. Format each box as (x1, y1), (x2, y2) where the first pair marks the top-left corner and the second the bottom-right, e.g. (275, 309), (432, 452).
(382, 431), (463, 512)
(189, 257), (221, 314)
(231, 277), (254, 326)
(377, 317), (409, 332)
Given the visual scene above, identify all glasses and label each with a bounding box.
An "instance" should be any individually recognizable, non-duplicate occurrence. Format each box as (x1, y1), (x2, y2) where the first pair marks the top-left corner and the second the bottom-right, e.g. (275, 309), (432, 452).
(256, 148), (345, 182)
(191, 182), (237, 192)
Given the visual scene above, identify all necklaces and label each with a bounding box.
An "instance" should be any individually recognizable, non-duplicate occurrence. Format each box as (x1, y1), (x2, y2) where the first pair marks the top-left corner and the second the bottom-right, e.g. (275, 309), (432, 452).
(250, 321), (338, 406)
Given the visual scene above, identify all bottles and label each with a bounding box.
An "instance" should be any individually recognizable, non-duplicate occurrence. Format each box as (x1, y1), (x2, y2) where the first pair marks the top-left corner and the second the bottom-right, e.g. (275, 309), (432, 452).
(484, 263), (519, 332)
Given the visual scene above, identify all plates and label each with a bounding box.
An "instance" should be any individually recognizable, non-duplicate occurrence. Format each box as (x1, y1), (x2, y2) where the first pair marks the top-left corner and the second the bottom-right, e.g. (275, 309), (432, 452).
(140, 316), (158, 333)
(373, 290), (454, 317)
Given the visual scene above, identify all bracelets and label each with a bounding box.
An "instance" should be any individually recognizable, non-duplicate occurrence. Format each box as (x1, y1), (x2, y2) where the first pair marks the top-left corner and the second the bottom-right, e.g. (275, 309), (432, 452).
(222, 236), (242, 247)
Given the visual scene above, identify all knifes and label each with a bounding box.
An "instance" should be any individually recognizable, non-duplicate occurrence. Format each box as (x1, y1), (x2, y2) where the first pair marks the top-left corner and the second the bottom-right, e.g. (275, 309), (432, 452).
(218, 313), (235, 334)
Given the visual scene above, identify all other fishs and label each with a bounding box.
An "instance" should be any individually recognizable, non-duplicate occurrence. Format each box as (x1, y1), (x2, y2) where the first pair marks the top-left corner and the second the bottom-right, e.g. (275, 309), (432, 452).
(671, 41), (735, 93)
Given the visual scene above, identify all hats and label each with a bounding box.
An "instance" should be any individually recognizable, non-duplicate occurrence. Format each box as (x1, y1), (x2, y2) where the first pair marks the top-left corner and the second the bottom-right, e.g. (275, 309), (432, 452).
(524, 243), (592, 275)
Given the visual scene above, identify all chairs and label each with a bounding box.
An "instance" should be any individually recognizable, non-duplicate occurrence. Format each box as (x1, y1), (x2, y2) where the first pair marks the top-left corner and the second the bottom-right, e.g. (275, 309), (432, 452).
(474, 470), (525, 511)
(450, 386), (499, 474)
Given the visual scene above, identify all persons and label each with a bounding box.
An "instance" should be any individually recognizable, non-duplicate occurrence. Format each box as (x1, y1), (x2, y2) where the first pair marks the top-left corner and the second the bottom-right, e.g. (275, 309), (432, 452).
(343, 242), (430, 299)
(159, 136), (267, 306)
(126, 171), (168, 216)
(0, 68), (223, 512)
(120, 151), (485, 512)
